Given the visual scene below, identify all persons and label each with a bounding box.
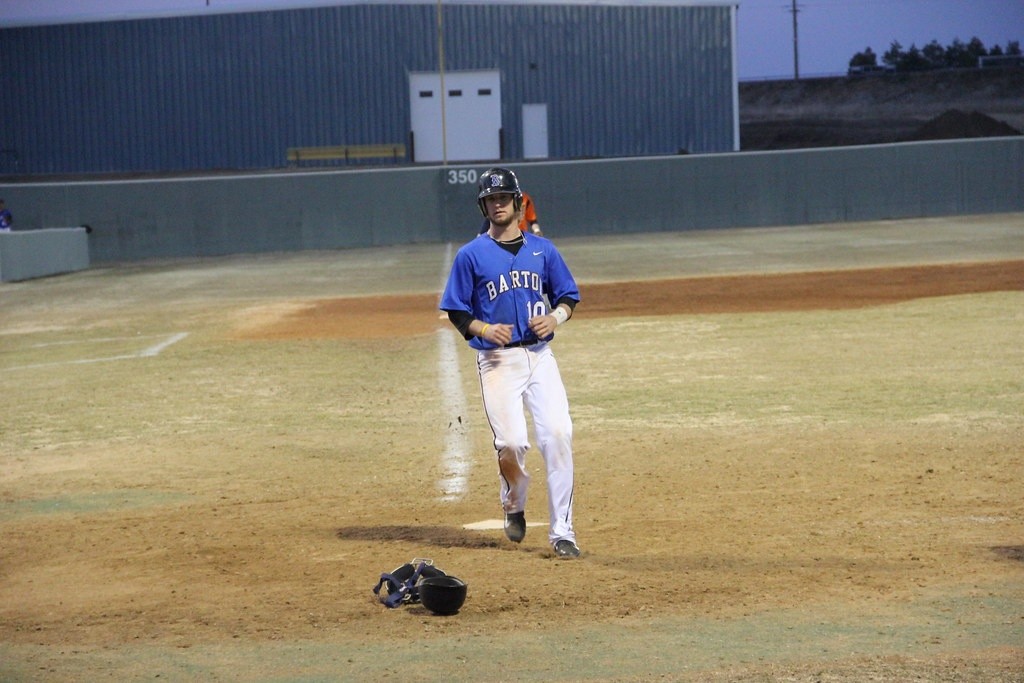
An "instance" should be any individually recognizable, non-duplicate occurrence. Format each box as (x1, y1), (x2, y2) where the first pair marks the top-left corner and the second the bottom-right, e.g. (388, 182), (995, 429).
(477, 192), (543, 238)
(440, 167), (581, 558)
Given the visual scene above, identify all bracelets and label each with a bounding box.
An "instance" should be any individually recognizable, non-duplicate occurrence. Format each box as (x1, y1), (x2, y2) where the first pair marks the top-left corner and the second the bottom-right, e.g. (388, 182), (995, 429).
(550, 306), (567, 326)
(481, 324), (489, 337)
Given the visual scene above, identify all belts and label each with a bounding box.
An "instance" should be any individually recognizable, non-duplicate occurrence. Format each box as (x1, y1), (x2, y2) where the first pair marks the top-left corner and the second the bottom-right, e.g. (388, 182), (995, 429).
(504, 339), (537, 347)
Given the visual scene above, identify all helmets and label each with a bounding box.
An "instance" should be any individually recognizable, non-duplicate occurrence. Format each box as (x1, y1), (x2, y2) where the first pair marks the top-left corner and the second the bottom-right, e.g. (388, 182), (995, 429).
(418, 576), (467, 615)
(478, 168), (523, 217)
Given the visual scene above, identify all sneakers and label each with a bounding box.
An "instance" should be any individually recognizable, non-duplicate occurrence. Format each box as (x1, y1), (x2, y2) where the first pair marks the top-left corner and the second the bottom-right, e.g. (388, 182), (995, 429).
(504, 510), (526, 543)
(553, 540), (579, 558)
